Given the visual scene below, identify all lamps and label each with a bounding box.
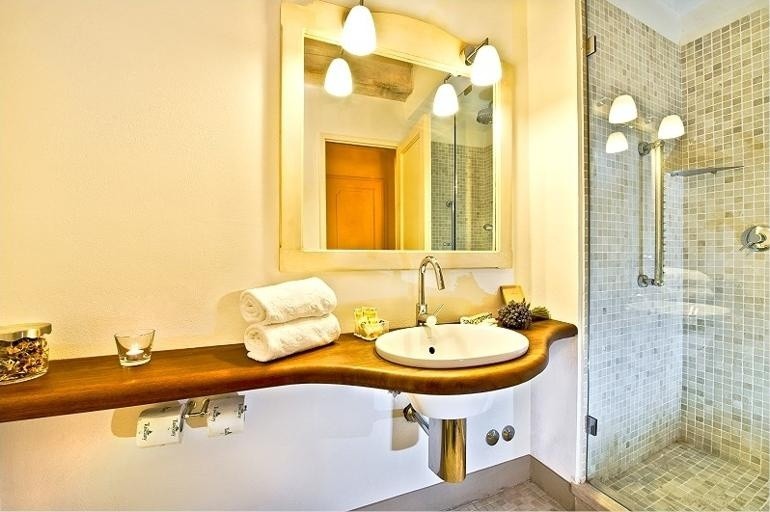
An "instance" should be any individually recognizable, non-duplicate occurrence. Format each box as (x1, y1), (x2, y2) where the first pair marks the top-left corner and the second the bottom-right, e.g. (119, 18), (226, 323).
(456, 35), (503, 89)
(323, 48), (355, 100)
(338, 1), (378, 58)
(603, 95), (637, 156)
(656, 114), (687, 141)
(432, 73), (460, 119)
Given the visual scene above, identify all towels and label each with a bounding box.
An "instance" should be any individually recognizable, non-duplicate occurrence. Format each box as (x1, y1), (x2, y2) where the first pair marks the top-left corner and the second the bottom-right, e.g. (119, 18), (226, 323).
(237, 274), (343, 362)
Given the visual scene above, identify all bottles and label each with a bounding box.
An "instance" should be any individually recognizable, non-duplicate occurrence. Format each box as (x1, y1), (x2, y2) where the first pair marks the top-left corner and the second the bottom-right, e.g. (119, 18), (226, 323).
(0, 320), (52, 387)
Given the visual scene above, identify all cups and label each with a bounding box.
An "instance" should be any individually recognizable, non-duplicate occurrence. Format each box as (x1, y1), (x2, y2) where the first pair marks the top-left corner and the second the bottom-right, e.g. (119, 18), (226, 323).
(113, 327), (156, 369)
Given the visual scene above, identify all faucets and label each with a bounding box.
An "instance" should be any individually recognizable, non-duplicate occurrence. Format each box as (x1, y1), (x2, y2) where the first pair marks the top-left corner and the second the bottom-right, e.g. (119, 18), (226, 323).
(419, 256), (445, 303)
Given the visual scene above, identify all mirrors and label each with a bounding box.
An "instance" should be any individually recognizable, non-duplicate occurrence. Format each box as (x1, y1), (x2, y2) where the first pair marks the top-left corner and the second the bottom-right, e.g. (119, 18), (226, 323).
(277, 1), (518, 276)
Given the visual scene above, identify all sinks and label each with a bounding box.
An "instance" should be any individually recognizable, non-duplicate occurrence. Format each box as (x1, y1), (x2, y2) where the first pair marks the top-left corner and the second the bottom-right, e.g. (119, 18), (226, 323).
(374, 325), (530, 369)
